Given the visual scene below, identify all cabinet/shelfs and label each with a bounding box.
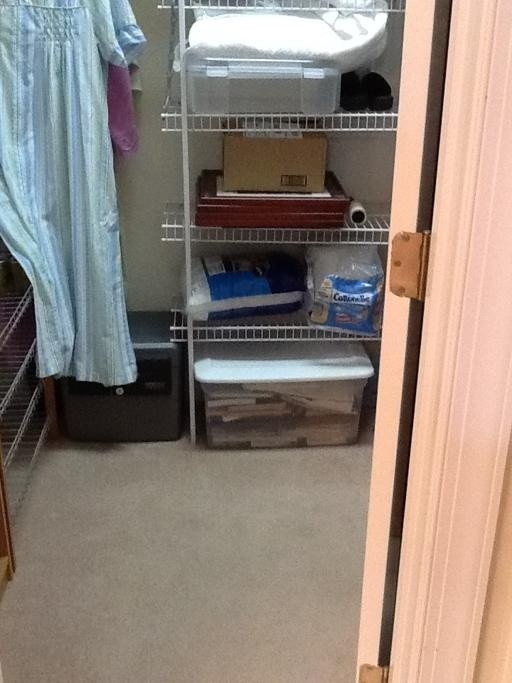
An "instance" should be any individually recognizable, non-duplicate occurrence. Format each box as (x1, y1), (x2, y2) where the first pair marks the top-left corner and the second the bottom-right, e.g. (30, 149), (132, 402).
(161, 0), (409, 448)
(0, 253), (61, 602)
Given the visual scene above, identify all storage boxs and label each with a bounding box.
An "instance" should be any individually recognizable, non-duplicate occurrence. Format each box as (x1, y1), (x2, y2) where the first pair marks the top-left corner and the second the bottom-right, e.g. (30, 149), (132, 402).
(57, 309), (185, 441)
(187, 57), (344, 116)
(193, 343), (374, 444)
(226, 134), (327, 193)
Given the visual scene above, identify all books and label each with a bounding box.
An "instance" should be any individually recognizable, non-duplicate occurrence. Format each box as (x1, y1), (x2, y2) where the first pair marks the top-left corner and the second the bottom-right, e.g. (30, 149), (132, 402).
(204, 379), (360, 449)
